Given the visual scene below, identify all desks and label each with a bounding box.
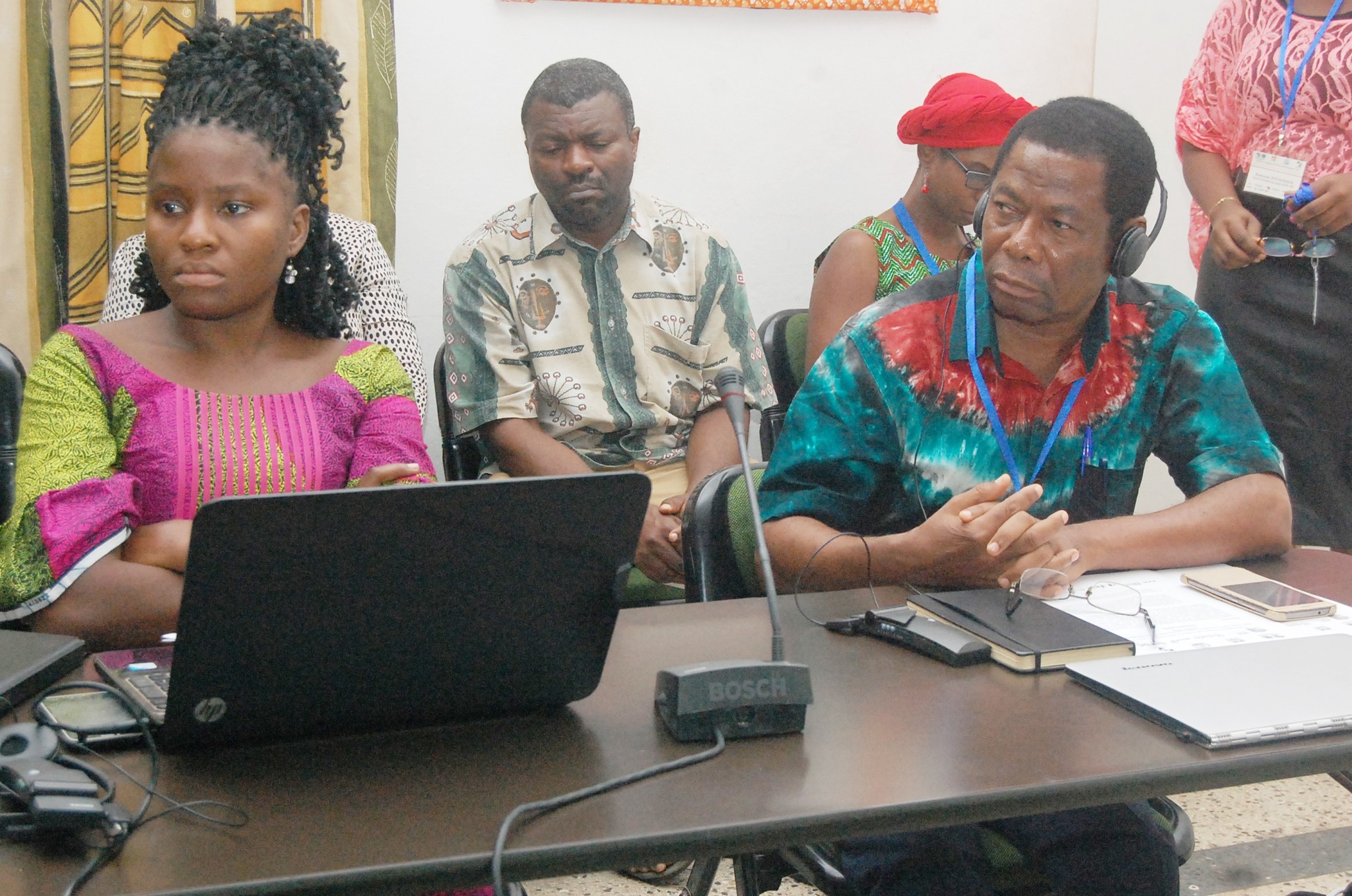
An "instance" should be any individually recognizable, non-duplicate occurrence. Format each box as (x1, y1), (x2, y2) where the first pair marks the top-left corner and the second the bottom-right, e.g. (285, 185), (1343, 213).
(2, 551), (1352, 896)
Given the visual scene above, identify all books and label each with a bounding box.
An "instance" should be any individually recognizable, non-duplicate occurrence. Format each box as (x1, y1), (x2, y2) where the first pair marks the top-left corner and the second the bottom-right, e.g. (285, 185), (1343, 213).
(907, 590), (1136, 672)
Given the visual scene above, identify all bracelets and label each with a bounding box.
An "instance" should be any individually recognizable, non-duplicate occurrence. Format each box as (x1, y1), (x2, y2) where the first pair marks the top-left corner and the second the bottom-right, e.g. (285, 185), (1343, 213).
(1208, 197), (1239, 219)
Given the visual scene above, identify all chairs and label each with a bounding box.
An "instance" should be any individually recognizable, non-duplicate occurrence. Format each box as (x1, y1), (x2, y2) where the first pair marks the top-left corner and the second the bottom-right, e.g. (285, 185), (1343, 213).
(431, 305), (825, 606)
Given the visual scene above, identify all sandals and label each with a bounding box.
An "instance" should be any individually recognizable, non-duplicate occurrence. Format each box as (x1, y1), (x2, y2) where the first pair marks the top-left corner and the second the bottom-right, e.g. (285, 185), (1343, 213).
(624, 860), (693, 882)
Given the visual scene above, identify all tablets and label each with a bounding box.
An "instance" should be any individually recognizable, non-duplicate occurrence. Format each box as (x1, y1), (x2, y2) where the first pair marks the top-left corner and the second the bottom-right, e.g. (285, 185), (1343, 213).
(37, 691), (144, 750)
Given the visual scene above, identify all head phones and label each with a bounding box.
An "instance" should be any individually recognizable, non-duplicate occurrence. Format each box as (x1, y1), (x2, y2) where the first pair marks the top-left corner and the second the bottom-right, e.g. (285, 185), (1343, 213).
(973, 168), (1167, 281)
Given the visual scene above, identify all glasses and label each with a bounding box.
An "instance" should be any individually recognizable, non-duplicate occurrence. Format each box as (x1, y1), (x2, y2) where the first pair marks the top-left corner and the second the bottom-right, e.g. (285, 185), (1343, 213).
(941, 148), (991, 190)
(1006, 568), (1155, 644)
(1256, 209), (1339, 258)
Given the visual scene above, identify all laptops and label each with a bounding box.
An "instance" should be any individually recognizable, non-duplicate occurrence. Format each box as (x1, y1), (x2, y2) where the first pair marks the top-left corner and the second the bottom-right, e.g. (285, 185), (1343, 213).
(92, 471), (651, 750)
(0, 628), (87, 713)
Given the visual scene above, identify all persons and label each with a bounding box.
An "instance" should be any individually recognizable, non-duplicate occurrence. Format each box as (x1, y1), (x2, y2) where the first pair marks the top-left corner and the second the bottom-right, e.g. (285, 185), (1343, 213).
(804, 72), (1037, 373)
(1175, 0), (1352, 555)
(441, 57), (779, 881)
(752, 98), (1292, 895)
(0, 12), (436, 649)
(106, 215), (429, 425)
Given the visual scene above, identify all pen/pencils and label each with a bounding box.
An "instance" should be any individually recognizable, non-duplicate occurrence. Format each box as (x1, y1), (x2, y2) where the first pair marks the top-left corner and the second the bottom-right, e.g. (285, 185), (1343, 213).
(1098, 458), (1108, 488)
(1080, 426), (1093, 476)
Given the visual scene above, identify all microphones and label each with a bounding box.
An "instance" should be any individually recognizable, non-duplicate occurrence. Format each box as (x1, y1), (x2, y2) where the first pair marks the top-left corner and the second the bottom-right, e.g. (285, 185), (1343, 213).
(657, 365), (811, 742)
